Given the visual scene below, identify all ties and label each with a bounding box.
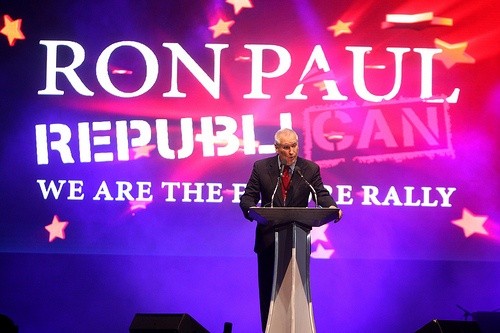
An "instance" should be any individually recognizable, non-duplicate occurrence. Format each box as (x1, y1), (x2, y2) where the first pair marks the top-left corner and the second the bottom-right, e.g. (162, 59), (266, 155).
(281, 166), (292, 202)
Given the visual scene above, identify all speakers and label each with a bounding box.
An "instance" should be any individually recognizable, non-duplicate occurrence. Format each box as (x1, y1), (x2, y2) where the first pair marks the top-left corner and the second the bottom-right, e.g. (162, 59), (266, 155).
(129, 313), (210, 333)
(0, 314), (18, 333)
(414, 319), (481, 333)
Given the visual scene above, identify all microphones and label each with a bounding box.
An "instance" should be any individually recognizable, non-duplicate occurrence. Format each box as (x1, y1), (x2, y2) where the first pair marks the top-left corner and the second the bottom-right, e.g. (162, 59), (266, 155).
(294, 165), (317, 208)
(271, 158), (287, 208)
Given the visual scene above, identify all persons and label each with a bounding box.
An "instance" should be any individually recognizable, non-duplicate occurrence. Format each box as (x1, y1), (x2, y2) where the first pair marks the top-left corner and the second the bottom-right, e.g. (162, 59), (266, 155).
(239, 128), (342, 333)
